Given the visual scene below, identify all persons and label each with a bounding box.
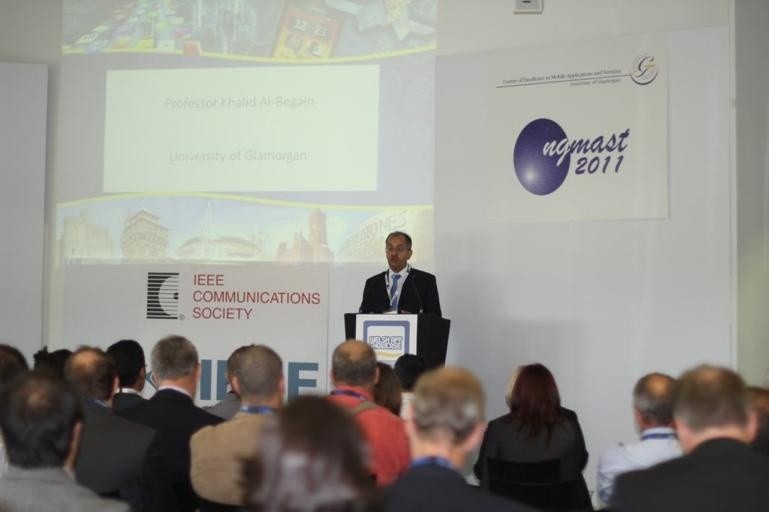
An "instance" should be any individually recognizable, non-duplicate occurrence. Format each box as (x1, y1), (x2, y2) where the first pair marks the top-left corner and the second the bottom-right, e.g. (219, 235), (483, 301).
(1, 333), (596, 512)
(596, 365), (769, 511)
(355, 229), (445, 318)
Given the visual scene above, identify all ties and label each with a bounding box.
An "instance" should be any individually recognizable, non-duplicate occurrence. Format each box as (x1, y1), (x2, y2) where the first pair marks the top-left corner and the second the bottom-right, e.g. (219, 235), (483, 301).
(388, 275), (400, 310)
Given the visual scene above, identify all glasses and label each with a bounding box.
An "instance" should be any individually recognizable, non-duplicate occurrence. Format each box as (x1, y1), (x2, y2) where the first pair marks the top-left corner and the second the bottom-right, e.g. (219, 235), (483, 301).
(385, 246), (408, 252)
